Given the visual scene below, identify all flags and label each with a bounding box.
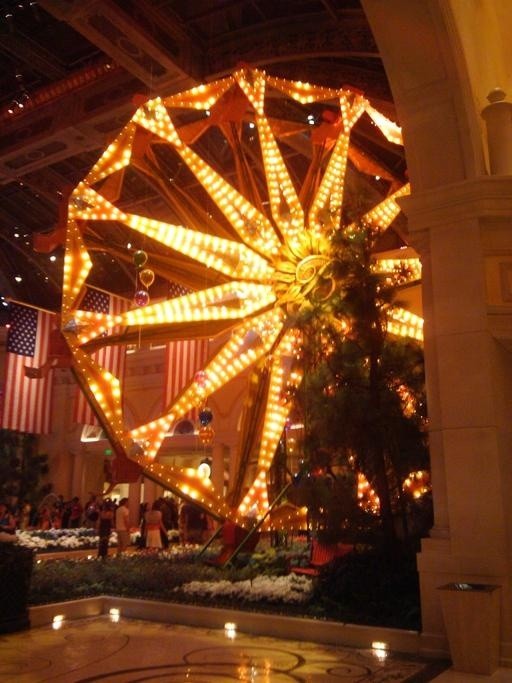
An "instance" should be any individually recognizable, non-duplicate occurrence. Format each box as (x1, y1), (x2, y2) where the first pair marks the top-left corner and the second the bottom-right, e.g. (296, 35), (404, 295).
(74, 285), (126, 427)
(0, 301), (58, 437)
(165, 279), (209, 431)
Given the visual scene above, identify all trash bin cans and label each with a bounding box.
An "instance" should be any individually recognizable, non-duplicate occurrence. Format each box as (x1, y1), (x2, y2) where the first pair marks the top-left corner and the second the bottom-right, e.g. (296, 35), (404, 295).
(435, 582), (501, 675)
(0, 544), (32, 636)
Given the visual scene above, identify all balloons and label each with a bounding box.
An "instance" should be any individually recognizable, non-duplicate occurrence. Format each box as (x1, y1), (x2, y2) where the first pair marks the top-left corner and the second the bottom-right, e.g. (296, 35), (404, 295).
(270, 438), (303, 508)
(133, 248), (157, 307)
(195, 370), (215, 449)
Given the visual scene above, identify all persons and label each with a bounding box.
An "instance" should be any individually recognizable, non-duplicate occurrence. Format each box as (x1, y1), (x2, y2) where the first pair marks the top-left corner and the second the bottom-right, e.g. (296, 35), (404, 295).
(1, 483), (212, 558)
